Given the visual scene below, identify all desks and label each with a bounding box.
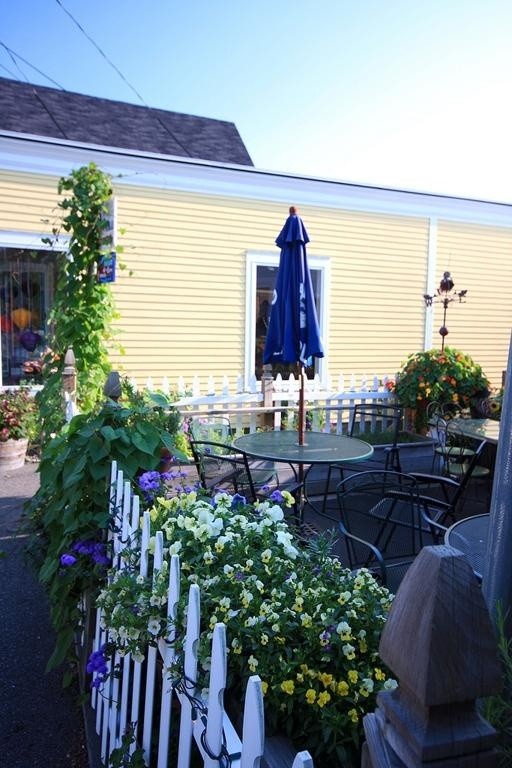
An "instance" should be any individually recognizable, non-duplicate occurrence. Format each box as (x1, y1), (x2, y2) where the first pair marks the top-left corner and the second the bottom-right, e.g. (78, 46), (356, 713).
(427, 419), (500, 513)
(231, 431), (374, 538)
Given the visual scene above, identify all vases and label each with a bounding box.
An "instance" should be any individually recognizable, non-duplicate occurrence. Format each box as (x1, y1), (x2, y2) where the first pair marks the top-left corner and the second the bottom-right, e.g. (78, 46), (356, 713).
(0, 438), (29, 471)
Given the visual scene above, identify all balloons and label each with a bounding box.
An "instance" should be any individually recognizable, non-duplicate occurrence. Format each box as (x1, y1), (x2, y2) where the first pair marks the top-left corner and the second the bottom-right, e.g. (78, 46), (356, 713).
(1, 315), (12, 331)
(21, 332), (38, 351)
(12, 309), (30, 330)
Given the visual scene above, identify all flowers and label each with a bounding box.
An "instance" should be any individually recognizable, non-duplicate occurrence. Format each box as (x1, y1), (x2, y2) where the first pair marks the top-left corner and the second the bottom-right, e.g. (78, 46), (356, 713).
(0, 387), (43, 442)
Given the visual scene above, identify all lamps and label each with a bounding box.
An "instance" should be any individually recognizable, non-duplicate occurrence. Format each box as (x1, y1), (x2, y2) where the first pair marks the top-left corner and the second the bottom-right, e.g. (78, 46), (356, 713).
(423, 271), (468, 352)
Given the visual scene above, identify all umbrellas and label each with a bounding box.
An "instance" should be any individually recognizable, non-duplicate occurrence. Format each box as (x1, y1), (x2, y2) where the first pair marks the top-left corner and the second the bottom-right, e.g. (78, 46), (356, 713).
(263, 206), (324, 539)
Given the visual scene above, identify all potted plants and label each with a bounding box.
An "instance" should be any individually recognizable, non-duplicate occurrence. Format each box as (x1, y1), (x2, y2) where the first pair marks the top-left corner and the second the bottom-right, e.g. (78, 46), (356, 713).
(20, 393), (191, 706)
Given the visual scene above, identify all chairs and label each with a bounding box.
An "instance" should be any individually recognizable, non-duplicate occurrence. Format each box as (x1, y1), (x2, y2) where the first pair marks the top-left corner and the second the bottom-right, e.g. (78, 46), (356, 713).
(435, 417), (490, 478)
(189, 416), (279, 494)
(190, 441), (304, 526)
(336, 469), (476, 596)
(426, 400), (476, 488)
(321, 403), (403, 513)
(364, 438), (487, 568)
